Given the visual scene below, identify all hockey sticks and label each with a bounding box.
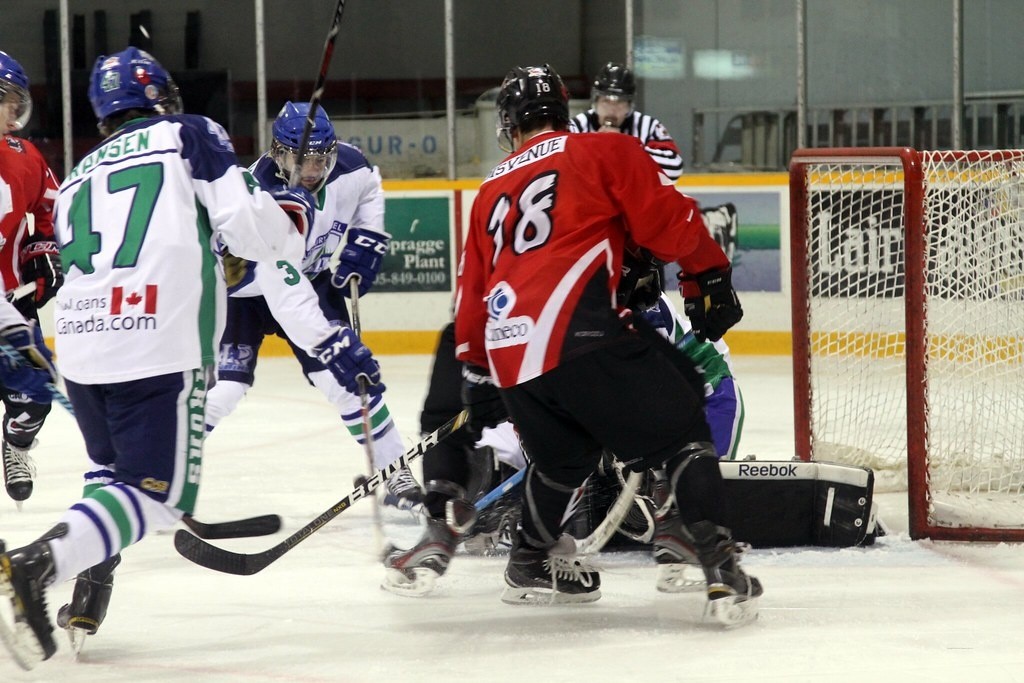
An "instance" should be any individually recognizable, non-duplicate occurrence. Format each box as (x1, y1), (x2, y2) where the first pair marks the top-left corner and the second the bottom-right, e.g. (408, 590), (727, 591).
(349, 277), (379, 496)
(287, 0), (347, 192)
(174, 410), (469, 578)
(475, 328), (702, 511)
(0, 341), (283, 540)
(377, 470), (643, 558)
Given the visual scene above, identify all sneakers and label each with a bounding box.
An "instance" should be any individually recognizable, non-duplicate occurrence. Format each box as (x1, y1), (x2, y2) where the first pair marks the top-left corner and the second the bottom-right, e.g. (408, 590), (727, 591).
(383, 464), (422, 499)
(0, 522), (70, 672)
(498, 516), (602, 606)
(56, 550), (122, 662)
(681, 518), (763, 629)
(376, 517), (458, 598)
(2, 437), (40, 513)
(651, 467), (707, 594)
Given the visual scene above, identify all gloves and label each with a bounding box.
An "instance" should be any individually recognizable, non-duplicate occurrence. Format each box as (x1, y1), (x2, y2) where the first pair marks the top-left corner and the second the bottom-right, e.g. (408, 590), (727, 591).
(331, 226), (393, 301)
(315, 319), (387, 396)
(18, 229), (64, 309)
(675, 261), (743, 344)
(460, 361), (493, 444)
(216, 234), (256, 294)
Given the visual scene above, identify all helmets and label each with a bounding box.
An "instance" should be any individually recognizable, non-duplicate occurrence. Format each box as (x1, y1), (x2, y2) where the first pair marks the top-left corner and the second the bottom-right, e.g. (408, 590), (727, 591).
(270, 100), (339, 156)
(0, 50), (33, 135)
(88, 45), (184, 136)
(591, 61), (638, 119)
(494, 62), (570, 154)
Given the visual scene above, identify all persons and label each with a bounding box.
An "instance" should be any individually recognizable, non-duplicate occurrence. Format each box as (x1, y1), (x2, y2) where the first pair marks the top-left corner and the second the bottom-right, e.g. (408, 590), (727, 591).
(0, 47), (385, 672)
(375, 60), (764, 631)
(205, 100), (424, 527)
(0, 49), (65, 508)
(569, 60), (685, 184)
